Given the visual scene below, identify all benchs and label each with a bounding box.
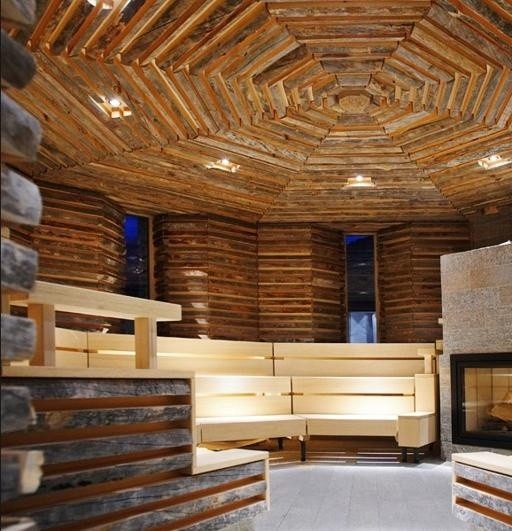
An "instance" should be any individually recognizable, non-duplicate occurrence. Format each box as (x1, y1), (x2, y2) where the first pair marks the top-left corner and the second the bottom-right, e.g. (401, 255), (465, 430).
(13, 281), (181, 368)
(451, 452), (512, 531)
